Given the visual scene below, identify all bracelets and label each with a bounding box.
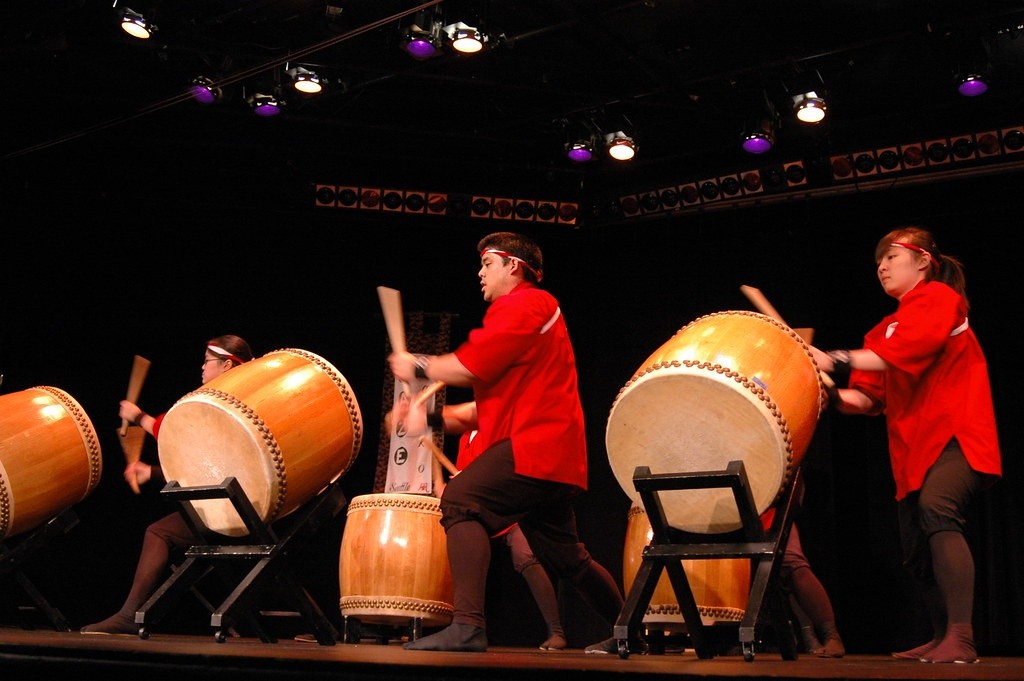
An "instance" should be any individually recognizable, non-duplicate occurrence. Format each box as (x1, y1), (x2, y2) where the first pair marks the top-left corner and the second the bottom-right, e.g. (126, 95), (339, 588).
(427, 402), (443, 428)
(831, 350), (852, 372)
(414, 355), (429, 380)
(136, 412), (145, 426)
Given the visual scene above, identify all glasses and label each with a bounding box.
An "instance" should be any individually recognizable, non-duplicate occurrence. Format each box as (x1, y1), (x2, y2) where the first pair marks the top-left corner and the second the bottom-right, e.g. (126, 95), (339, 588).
(204, 358), (223, 364)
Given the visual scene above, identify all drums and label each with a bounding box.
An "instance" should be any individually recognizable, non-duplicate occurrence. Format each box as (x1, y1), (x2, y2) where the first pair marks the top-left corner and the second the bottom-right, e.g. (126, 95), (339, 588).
(620, 499), (754, 629)
(1, 384), (104, 540)
(156, 347), (365, 538)
(336, 492), (453, 629)
(603, 309), (824, 536)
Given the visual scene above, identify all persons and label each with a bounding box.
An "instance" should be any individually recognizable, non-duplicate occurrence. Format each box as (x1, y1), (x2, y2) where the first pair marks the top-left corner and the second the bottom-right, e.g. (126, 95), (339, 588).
(382, 231), (647, 654)
(759, 506), (845, 658)
(79, 336), (323, 643)
(804, 227), (1002, 662)
